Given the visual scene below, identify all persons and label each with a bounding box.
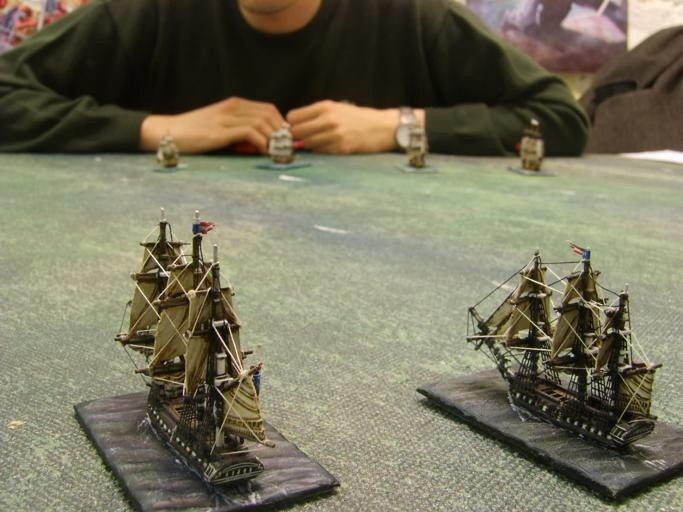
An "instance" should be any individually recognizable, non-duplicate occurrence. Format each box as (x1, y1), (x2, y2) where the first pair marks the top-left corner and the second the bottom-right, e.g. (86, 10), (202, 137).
(1, 0), (590, 159)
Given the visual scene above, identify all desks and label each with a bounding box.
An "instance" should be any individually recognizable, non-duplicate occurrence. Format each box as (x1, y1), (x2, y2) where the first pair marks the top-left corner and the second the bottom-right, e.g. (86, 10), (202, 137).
(1, 143), (682, 512)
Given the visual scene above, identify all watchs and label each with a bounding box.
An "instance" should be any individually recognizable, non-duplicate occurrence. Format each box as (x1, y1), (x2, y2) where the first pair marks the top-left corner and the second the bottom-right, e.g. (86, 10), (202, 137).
(395, 105), (419, 152)
